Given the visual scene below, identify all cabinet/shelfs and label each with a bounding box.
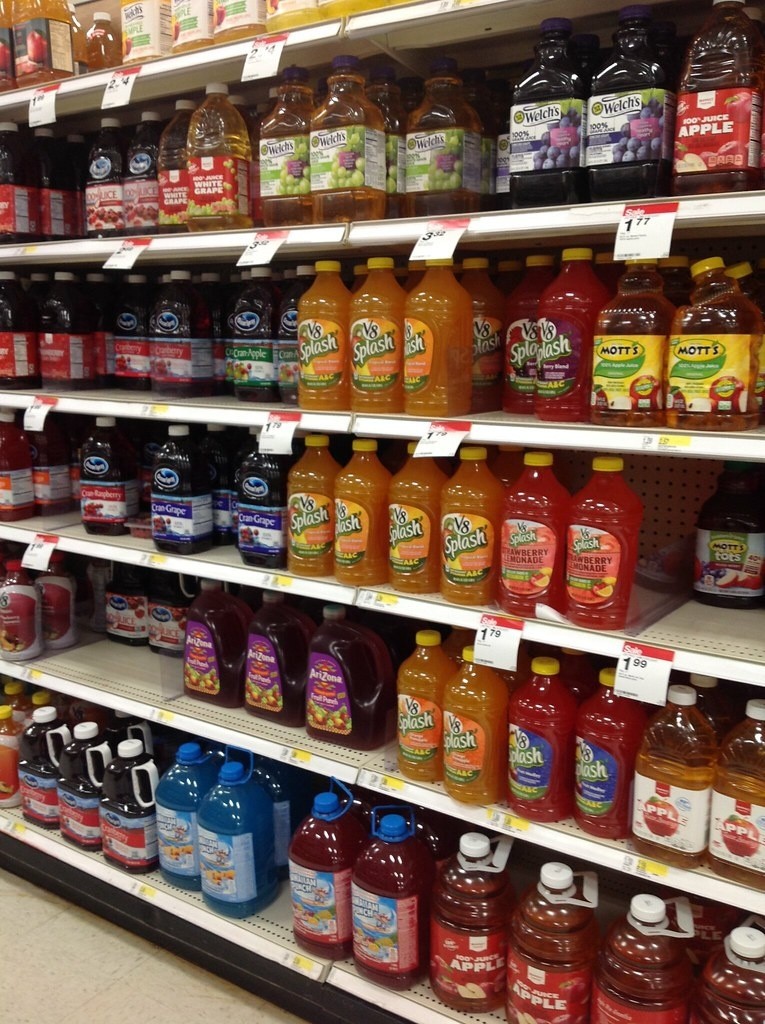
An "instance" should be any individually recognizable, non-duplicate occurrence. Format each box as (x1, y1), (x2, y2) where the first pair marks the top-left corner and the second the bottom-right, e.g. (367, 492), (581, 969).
(0, 0), (765, 1024)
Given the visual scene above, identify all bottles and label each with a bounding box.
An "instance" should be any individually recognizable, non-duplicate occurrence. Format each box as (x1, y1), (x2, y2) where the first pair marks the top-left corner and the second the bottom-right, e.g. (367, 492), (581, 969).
(0, 247), (765, 432)
(0, 0), (765, 245)
(0, 549), (765, 894)
(0, 409), (765, 631)
(0, 675), (765, 1024)
(0, 1), (414, 92)
